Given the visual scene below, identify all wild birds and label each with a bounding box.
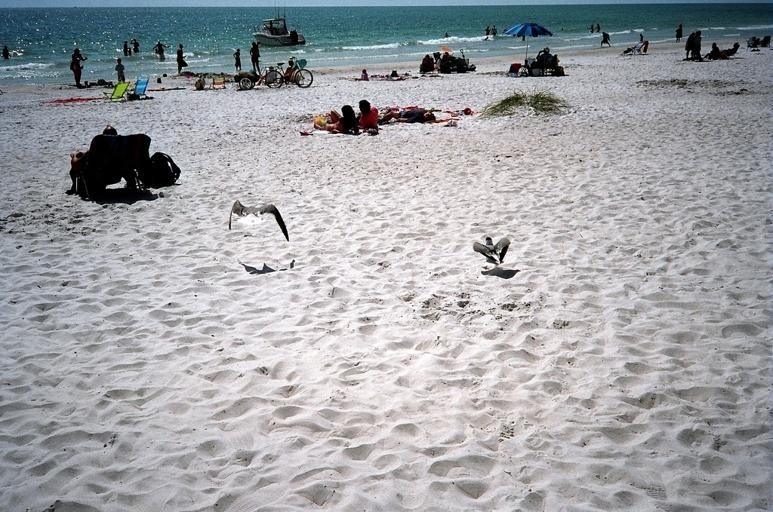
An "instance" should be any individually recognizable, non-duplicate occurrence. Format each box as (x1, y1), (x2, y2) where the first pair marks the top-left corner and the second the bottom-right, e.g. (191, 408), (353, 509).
(229, 200), (290, 242)
(474, 236), (510, 266)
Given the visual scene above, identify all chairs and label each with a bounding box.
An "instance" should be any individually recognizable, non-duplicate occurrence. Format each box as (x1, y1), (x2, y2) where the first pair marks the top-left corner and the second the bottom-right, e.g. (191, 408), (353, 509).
(746, 35), (771, 49)
(520, 54), (559, 77)
(623, 41), (648, 56)
(705, 43), (739, 61)
(103, 78), (149, 103)
(209, 76), (226, 91)
(71, 135), (151, 201)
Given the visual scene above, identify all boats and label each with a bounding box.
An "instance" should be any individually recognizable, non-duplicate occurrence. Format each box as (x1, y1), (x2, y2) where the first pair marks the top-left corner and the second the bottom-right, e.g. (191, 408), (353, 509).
(250, 7), (306, 46)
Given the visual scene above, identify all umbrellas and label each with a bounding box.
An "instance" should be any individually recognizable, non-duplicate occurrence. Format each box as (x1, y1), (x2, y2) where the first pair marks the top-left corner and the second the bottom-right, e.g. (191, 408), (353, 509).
(503, 22), (552, 59)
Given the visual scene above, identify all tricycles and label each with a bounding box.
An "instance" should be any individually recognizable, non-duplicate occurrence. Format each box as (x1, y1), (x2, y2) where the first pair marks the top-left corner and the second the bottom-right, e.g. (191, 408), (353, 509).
(233, 65), (276, 90)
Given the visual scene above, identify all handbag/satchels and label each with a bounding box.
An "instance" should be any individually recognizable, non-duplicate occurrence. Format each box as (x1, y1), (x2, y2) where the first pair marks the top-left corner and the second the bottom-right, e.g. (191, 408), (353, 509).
(138, 152), (180, 188)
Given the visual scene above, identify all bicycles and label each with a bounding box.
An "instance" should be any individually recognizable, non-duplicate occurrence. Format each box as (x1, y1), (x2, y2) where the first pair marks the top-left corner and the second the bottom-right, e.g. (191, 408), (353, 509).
(263, 55), (313, 88)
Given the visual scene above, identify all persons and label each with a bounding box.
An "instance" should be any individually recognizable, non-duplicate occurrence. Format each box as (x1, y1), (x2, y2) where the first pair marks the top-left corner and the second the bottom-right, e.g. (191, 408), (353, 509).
(443, 31), (448, 37)
(324, 105), (359, 135)
(684, 31), (760, 62)
(596, 24), (600, 32)
(360, 68), (367, 80)
(256, 66), (274, 86)
(175, 43), (188, 73)
(114, 57), (125, 81)
(248, 41), (260, 74)
(535, 46), (552, 66)
(232, 48), (241, 71)
(68, 123), (117, 168)
(122, 38), (169, 60)
(482, 26), (489, 40)
(420, 51), (467, 75)
(390, 68), (398, 76)
(490, 25), (497, 35)
(599, 31), (611, 47)
(71, 47), (87, 89)
(277, 59), (294, 83)
(355, 100), (378, 133)
(639, 33), (643, 42)
(2, 45), (10, 59)
(673, 24), (682, 43)
(377, 107), (435, 123)
(589, 24), (593, 33)
